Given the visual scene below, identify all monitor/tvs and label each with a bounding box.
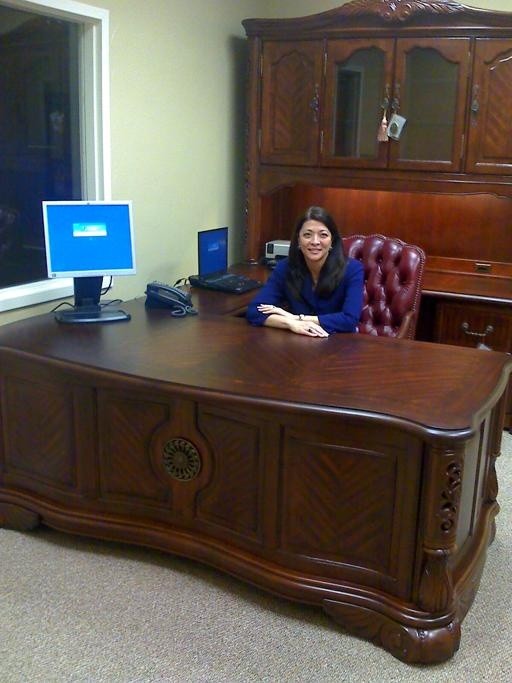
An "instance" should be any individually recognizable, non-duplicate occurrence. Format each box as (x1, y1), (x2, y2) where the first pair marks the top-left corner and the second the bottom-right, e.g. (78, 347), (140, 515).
(42, 201), (138, 324)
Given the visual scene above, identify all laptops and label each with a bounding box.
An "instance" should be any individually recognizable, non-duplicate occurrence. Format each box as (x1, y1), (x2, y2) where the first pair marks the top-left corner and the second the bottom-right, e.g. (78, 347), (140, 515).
(198, 227), (262, 294)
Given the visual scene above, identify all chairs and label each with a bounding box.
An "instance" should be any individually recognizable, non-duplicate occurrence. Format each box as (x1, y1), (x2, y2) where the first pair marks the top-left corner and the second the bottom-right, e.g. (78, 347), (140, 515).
(338, 234), (426, 341)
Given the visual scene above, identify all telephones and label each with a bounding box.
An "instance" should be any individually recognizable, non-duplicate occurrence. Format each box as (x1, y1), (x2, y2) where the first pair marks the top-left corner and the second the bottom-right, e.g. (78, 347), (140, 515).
(145, 283), (193, 309)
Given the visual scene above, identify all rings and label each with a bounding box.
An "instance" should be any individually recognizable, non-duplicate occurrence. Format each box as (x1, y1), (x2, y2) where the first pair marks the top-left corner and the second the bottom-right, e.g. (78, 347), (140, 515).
(273, 305), (275, 308)
(308, 327), (311, 333)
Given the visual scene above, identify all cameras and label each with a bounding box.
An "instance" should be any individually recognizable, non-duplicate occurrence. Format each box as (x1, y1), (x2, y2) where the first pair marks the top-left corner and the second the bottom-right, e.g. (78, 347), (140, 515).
(387, 112), (407, 142)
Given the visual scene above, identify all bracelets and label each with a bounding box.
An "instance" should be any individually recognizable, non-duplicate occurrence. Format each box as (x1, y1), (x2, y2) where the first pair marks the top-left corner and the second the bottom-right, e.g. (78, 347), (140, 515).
(299, 314), (305, 320)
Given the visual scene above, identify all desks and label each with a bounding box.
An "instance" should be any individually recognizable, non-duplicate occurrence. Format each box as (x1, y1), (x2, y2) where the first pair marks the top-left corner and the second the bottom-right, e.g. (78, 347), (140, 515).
(0, 262), (512, 665)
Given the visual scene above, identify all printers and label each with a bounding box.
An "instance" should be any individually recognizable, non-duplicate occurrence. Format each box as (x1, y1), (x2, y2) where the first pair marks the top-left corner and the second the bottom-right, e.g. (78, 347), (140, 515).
(265, 240), (292, 270)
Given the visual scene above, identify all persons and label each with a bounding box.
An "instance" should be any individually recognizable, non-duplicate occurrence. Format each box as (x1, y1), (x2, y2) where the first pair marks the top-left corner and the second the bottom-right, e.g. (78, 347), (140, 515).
(242, 205), (365, 337)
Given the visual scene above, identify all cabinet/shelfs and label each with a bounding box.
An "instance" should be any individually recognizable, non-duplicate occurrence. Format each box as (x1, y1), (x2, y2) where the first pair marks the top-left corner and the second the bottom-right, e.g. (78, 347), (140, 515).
(240, 0), (512, 439)
